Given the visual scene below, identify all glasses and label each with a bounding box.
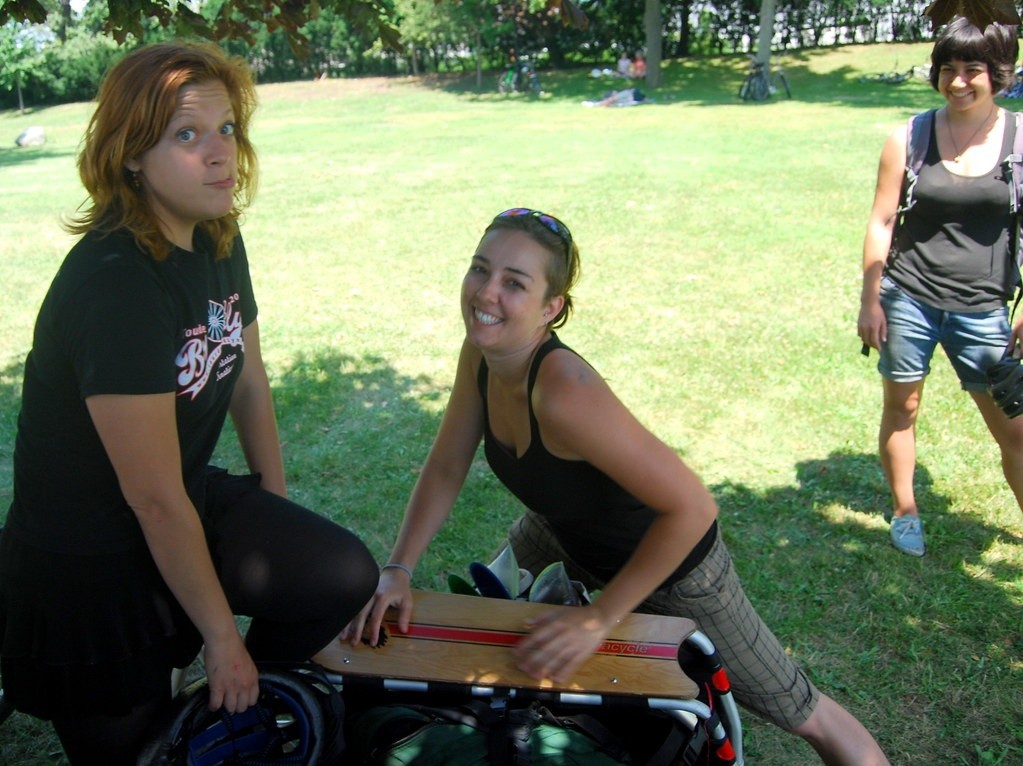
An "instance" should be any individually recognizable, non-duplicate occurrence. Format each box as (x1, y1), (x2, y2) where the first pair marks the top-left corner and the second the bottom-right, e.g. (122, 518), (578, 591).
(494, 208), (569, 264)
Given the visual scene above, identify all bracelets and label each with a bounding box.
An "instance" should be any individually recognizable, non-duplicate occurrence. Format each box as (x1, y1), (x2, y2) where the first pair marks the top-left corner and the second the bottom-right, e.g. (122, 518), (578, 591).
(383, 564), (412, 578)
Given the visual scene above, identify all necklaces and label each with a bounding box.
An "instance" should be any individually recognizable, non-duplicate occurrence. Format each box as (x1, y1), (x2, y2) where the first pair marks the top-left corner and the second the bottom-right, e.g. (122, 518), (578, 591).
(946, 104), (994, 162)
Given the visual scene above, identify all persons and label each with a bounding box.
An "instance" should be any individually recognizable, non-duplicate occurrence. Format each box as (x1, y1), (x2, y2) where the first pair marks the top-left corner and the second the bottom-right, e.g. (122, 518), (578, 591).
(616, 52), (631, 78)
(0, 41), (380, 766)
(857, 16), (1022, 554)
(596, 89), (642, 105)
(339, 207), (891, 766)
(632, 50), (647, 79)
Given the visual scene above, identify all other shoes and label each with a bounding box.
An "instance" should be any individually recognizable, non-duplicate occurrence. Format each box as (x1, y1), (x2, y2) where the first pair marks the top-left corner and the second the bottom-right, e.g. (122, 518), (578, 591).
(889, 513), (925, 557)
